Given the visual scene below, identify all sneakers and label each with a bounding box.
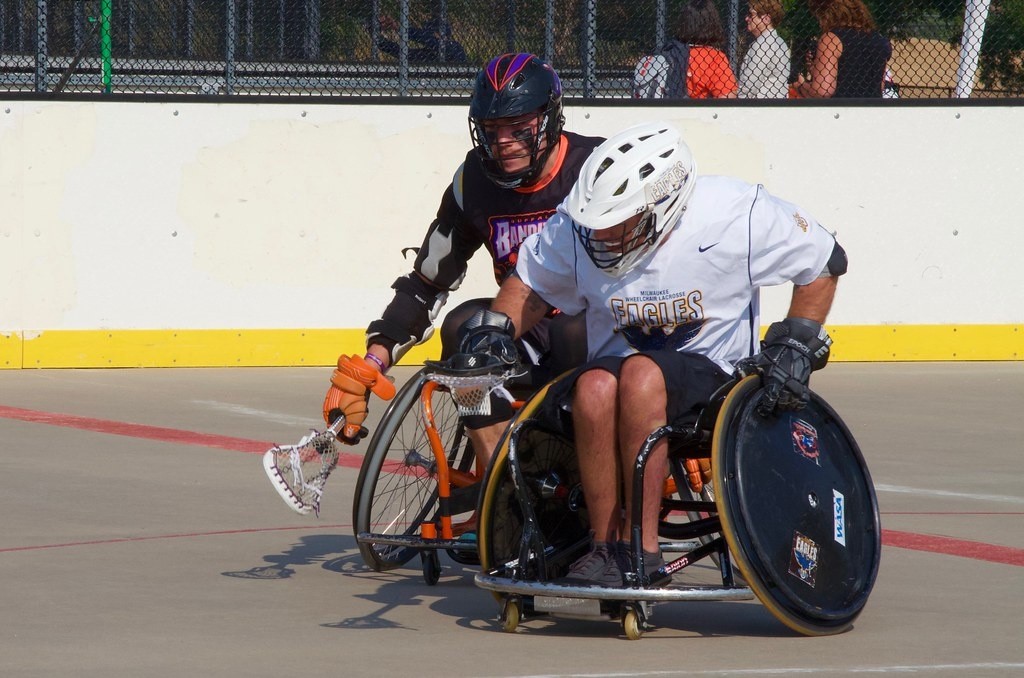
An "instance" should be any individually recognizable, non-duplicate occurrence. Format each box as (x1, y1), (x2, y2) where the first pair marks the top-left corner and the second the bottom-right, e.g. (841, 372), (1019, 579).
(597, 532), (672, 590)
(564, 530), (615, 582)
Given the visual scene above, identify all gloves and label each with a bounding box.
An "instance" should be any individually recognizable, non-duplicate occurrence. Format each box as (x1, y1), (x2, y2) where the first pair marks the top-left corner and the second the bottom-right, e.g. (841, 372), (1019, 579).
(323, 354), (397, 446)
(731, 317), (834, 420)
(684, 457), (713, 494)
(445, 308), (520, 380)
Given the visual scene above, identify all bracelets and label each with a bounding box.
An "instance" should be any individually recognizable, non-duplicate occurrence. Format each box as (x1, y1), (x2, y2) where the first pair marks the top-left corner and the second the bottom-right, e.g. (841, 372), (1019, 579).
(366, 352), (386, 372)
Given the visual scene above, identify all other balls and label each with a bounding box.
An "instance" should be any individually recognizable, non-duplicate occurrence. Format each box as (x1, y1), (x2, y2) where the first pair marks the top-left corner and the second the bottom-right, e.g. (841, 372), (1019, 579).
(453, 384), (485, 409)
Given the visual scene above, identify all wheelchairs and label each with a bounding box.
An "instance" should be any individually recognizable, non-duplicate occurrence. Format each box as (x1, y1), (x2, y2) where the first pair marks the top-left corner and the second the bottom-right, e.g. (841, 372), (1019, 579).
(353, 364), (884, 640)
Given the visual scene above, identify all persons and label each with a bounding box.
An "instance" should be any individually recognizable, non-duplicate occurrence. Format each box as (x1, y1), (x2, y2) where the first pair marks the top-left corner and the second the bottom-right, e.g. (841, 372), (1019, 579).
(798, 0), (900, 99)
(324, 54), (607, 482)
(673, 0), (738, 98)
(738, 0), (791, 99)
(452, 125), (848, 590)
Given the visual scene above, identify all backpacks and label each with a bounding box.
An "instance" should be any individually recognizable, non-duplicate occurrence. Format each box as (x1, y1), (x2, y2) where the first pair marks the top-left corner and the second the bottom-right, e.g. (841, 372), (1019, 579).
(631, 40), (705, 100)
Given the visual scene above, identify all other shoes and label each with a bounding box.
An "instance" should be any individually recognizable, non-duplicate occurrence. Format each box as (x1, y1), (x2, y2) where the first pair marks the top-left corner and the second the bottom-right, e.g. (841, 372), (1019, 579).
(449, 529), (478, 556)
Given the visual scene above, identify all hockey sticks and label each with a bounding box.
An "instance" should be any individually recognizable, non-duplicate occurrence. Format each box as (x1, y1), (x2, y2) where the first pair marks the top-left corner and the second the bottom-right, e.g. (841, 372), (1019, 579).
(421, 346), (536, 420)
(260, 413), (348, 520)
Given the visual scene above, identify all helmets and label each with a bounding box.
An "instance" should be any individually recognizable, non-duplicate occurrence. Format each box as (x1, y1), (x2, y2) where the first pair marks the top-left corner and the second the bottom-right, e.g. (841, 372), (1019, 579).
(556, 126), (696, 279)
(468, 54), (565, 190)
